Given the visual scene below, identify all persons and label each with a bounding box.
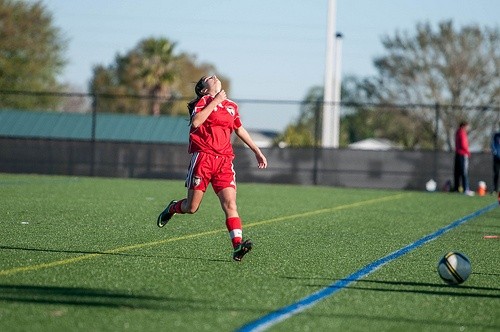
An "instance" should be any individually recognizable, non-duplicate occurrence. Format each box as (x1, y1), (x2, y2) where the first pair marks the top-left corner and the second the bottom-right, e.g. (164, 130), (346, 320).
(156, 74), (268, 262)
(491, 132), (500, 194)
(454, 119), (477, 195)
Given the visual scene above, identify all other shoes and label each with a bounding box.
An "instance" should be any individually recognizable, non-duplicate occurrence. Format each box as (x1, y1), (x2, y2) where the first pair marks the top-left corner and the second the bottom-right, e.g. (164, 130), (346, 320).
(464, 189), (474, 195)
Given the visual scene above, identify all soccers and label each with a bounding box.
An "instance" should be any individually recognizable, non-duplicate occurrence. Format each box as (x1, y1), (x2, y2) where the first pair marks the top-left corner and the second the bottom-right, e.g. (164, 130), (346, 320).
(437, 251), (471, 286)
(425, 179), (437, 192)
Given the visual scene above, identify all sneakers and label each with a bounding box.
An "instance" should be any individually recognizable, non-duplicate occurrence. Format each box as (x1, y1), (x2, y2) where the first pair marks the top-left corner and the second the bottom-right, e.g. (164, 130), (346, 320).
(233, 239), (252, 261)
(157, 200), (178, 227)
